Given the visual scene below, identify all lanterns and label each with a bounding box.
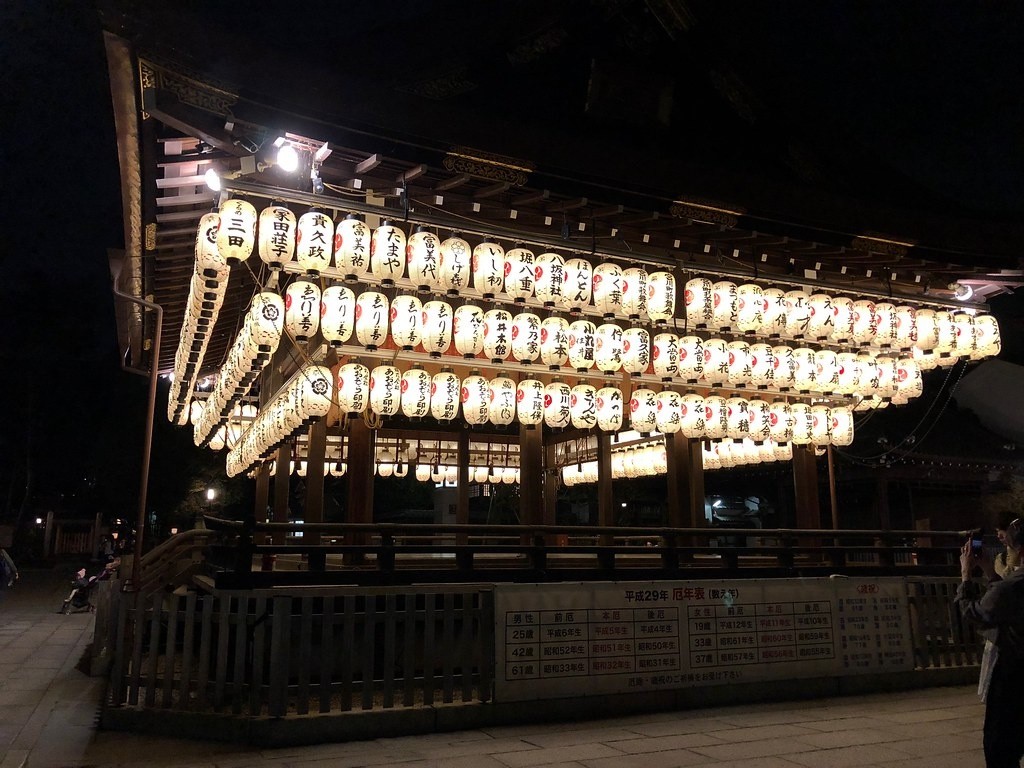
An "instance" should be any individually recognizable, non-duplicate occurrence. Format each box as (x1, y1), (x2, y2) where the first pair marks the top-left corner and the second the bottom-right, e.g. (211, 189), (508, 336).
(167, 199), (1003, 488)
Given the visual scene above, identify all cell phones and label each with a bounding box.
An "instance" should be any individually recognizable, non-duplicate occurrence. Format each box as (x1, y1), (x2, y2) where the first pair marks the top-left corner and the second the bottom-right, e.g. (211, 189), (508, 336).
(970, 532), (984, 560)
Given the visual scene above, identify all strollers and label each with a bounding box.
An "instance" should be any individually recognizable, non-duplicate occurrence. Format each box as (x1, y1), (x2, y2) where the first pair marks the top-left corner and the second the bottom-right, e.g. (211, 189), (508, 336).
(62, 569), (115, 616)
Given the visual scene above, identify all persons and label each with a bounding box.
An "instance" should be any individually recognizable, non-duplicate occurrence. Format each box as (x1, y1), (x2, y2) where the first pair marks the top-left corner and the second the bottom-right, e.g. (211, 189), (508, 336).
(0, 549), (18, 589)
(105, 552), (122, 576)
(959, 511), (1024, 768)
(64, 568), (88, 602)
